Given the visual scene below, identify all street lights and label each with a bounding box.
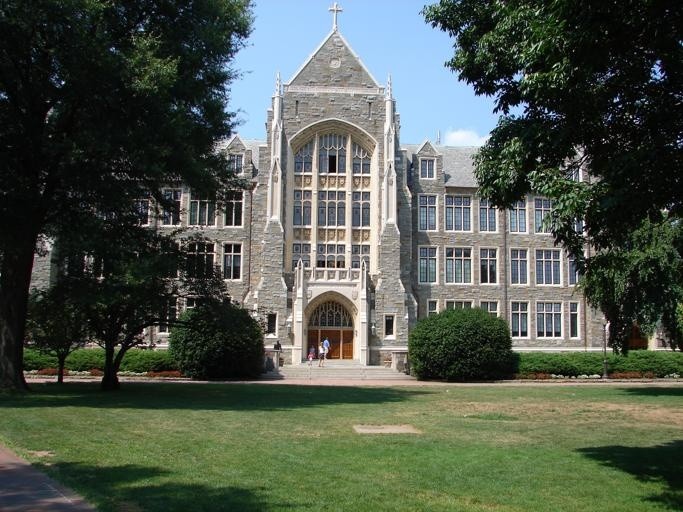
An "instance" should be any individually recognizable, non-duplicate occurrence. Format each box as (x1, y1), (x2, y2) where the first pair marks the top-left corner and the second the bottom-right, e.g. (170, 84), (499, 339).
(601, 319), (608, 379)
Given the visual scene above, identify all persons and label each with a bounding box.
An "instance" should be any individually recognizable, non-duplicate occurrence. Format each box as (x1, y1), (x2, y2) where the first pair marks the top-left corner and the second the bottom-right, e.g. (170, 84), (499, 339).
(307, 337), (331, 367)
(274, 340), (281, 350)
